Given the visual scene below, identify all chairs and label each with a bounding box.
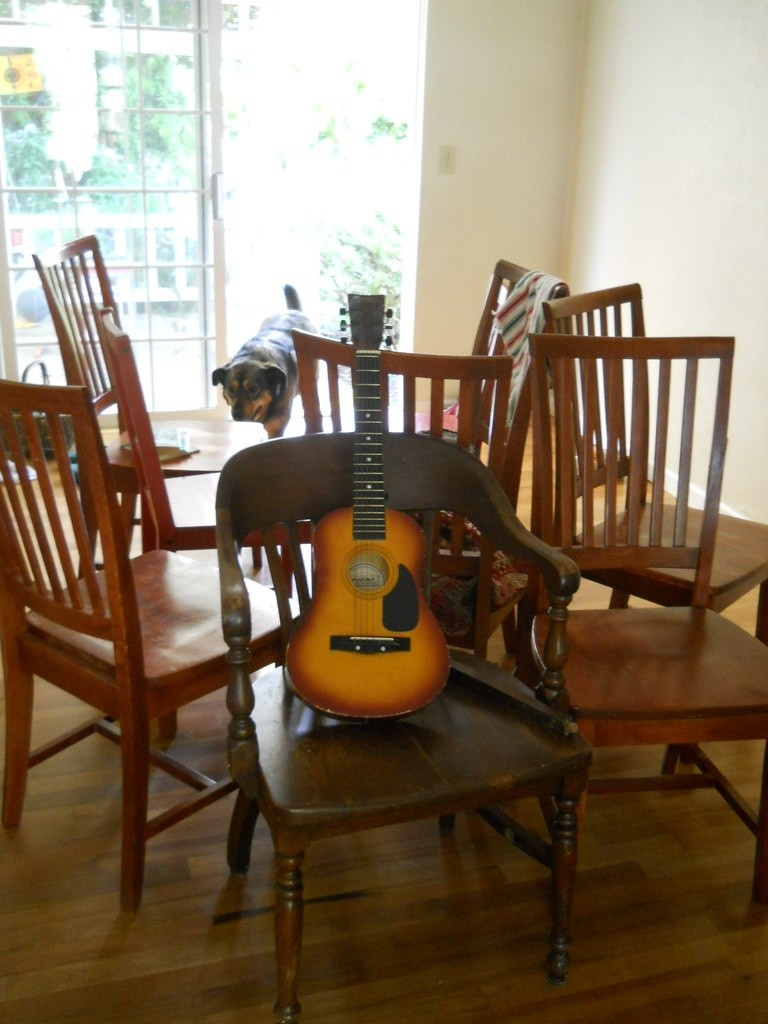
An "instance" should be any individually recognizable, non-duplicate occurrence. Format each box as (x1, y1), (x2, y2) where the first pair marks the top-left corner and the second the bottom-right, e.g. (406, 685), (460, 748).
(216, 432), (594, 1024)
(32, 235), (268, 577)
(0, 381), (301, 914)
(542, 283), (768, 764)
(454, 259), (571, 657)
(528, 334), (768, 904)
(289, 327), (528, 661)
(99, 304), (310, 737)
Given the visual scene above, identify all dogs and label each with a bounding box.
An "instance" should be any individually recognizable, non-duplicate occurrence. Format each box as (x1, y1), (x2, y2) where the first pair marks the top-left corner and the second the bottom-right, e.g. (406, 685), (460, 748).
(211, 284), (319, 441)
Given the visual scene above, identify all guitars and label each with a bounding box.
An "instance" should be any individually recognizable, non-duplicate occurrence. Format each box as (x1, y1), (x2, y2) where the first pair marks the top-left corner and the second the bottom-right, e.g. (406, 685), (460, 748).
(283, 294), (452, 723)
